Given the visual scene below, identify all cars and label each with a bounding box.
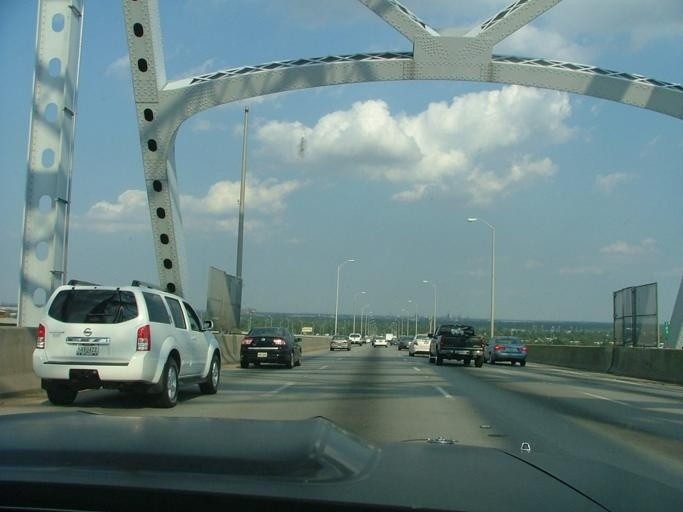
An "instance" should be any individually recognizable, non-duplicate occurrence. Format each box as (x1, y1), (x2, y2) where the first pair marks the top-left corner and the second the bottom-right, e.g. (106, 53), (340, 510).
(241, 326), (302, 369)
(484, 336), (528, 366)
(398, 336), (415, 351)
(329, 335), (352, 352)
(349, 333), (399, 348)
(409, 334), (433, 356)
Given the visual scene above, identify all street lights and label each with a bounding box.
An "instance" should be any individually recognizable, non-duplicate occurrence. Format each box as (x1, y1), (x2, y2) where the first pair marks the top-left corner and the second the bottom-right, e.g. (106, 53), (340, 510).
(335, 259), (356, 336)
(353, 291), (367, 334)
(422, 280), (437, 334)
(408, 300), (418, 336)
(360, 304), (409, 337)
(467, 217), (495, 338)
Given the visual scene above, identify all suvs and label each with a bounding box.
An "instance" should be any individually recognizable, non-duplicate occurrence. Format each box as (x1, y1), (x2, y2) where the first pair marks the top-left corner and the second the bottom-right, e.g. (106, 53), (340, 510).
(32, 280), (222, 408)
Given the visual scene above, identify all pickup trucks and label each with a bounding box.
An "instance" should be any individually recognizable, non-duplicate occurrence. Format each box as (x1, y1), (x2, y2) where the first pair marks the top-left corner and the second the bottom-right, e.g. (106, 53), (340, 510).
(428, 323), (484, 368)
